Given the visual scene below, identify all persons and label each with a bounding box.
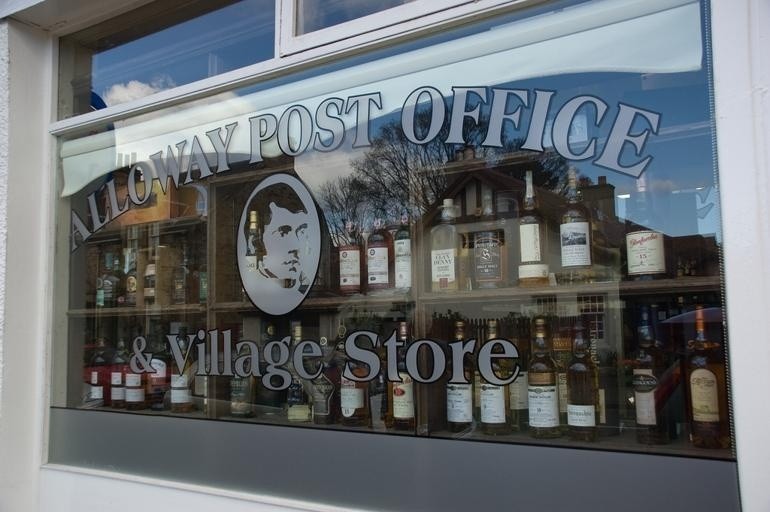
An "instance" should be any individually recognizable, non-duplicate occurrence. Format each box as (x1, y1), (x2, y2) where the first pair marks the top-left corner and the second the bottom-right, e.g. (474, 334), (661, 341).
(244, 183), (308, 306)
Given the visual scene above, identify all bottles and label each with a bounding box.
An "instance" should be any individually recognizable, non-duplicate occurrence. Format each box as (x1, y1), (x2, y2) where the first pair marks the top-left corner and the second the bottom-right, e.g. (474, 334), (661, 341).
(85, 320), (208, 416)
(224, 318), (416, 431)
(91, 223), (208, 308)
(234, 197), (414, 304)
(426, 164), (674, 297)
(438, 301), (727, 450)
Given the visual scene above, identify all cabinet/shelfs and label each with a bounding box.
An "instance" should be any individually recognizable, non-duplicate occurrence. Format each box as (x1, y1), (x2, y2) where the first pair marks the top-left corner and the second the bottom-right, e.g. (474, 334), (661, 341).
(67, 119), (738, 462)
